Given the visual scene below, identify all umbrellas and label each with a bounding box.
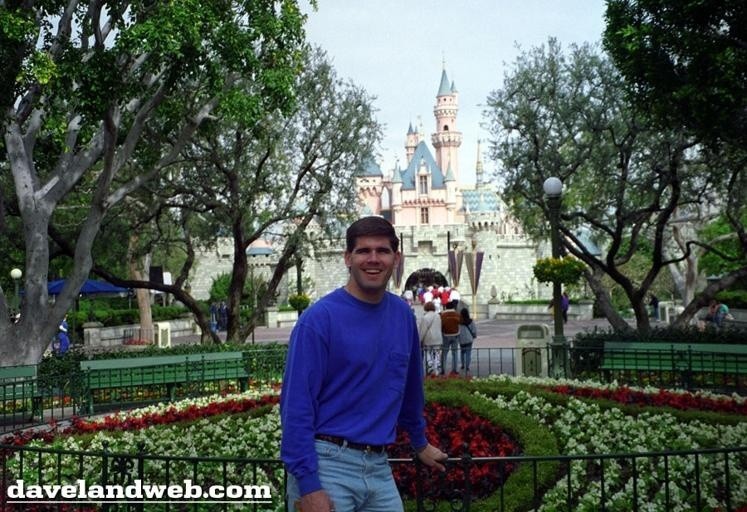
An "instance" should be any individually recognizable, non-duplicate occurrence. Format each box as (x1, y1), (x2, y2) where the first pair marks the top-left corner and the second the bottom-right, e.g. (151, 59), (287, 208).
(47, 277), (129, 353)
(19, 278), (59, 303)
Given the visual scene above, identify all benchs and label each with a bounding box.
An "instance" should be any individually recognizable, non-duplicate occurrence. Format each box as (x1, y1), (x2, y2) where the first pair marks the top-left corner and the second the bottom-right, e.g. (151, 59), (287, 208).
(0, 363), (45, 425)
(78, 350), (255, 416)
(595, 340), (747, 396)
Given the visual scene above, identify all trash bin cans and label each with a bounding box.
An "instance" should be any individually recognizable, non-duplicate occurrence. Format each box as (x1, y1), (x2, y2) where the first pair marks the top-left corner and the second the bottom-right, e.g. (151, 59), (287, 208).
(658, 301), (675, 325)
(514, 323), (553, 378)
(153, 322), (171, 349)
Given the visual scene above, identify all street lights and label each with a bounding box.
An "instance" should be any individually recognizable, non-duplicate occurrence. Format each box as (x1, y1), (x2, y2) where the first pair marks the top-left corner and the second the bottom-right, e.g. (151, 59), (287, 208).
(10, 268), (22, 324)
(543, 176), (568, 378)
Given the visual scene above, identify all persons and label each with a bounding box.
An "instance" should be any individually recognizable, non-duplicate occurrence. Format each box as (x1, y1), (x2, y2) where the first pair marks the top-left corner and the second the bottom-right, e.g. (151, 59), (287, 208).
(690, 298), (734, 333)
(561, 292), (569, 322)
(549, 293), (555, 308)
(399, 275), (477, 375)
(648, 294), (660, 321)
(280, 217), (449, 512)
(209, 301), (232, 330)
(59, 313), (70, 355)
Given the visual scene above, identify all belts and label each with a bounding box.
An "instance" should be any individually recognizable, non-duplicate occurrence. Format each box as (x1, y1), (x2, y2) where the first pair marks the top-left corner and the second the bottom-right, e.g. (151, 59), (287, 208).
(316, 434), (387, 453)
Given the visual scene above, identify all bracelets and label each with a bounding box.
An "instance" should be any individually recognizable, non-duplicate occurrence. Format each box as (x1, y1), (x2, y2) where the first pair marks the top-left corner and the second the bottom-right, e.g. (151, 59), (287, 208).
(416, 444), (427, 454)
